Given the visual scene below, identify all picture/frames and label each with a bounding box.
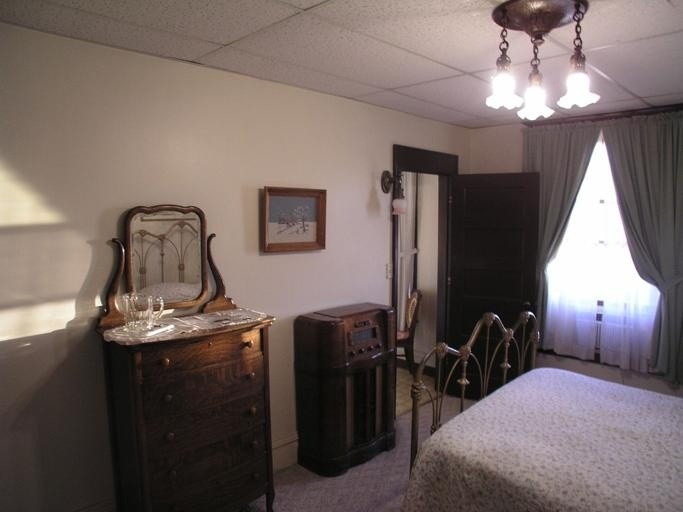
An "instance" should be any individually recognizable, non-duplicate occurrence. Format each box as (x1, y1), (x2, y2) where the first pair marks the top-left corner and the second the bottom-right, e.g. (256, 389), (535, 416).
(259, 185), (325, 252)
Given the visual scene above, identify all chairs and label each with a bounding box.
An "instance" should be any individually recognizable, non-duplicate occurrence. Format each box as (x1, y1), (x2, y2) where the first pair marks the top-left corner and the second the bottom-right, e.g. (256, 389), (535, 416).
(397, 288), (422, 376)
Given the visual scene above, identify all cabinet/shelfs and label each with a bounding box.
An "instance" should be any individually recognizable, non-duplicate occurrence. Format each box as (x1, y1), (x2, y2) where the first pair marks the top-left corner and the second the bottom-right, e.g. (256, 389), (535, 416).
(293, 301), (397, 479)
(102, 309), (275, 511)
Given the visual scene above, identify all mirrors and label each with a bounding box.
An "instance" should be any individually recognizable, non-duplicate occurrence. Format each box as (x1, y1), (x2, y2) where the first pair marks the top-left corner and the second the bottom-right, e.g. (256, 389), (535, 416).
(98, 204), (238, 328)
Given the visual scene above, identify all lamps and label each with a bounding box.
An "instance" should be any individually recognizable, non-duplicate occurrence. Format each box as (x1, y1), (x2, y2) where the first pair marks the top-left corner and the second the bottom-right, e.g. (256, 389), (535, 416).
(484, 0), (524, 111)
(519, 33), (554, 120)
(556, 0), (602, 110)
(381, 166), (408, 215)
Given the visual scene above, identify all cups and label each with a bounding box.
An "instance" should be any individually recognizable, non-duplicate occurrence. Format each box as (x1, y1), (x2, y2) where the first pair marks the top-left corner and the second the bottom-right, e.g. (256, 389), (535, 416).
(120, 292), (165, 332)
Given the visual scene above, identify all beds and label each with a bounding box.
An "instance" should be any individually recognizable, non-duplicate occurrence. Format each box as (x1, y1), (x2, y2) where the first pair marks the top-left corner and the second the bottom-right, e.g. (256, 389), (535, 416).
(413, 312), (683, 512)
